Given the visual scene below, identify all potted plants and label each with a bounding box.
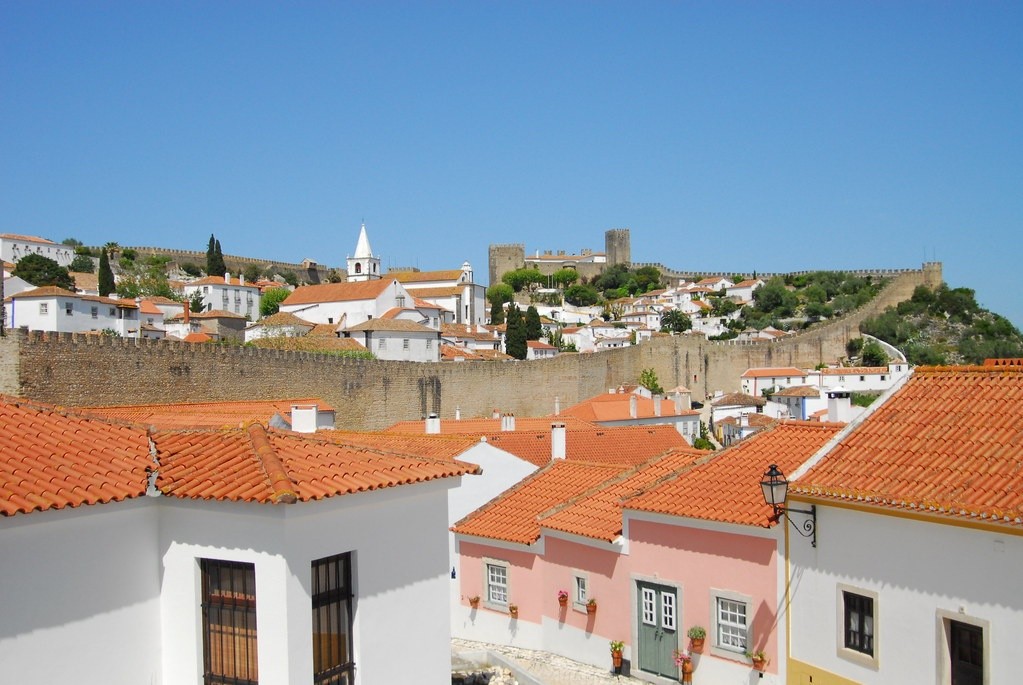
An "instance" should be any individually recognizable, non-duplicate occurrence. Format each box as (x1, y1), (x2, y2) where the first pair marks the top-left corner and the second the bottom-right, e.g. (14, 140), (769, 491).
(746, 650), (766, 670)
(687, 625), (706, 649)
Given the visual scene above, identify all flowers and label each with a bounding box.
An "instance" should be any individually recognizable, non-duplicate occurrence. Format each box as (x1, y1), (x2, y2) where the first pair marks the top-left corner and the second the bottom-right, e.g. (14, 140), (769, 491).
(557, 590), (568, 599)
(584, 595), (596, 606)
(468, 593), (480, 603)
(673, 649), (692, 667)
(608, 640), (625, 652)
(508, 603), (519, 612)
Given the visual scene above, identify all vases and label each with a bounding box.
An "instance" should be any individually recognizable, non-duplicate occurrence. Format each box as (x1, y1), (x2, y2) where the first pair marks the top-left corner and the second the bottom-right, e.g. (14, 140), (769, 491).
(558, 598), (567, 607)
(612, 651), (622, 667)
(682, 661), (692, 685)
(586, 604), (596, 612)
(471, 602), (478, 608)
(511, 610), (517, 618)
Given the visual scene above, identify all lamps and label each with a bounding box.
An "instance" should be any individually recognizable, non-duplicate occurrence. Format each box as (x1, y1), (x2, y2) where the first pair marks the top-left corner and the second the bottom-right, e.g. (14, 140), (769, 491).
(760, 464), (817, 548)
